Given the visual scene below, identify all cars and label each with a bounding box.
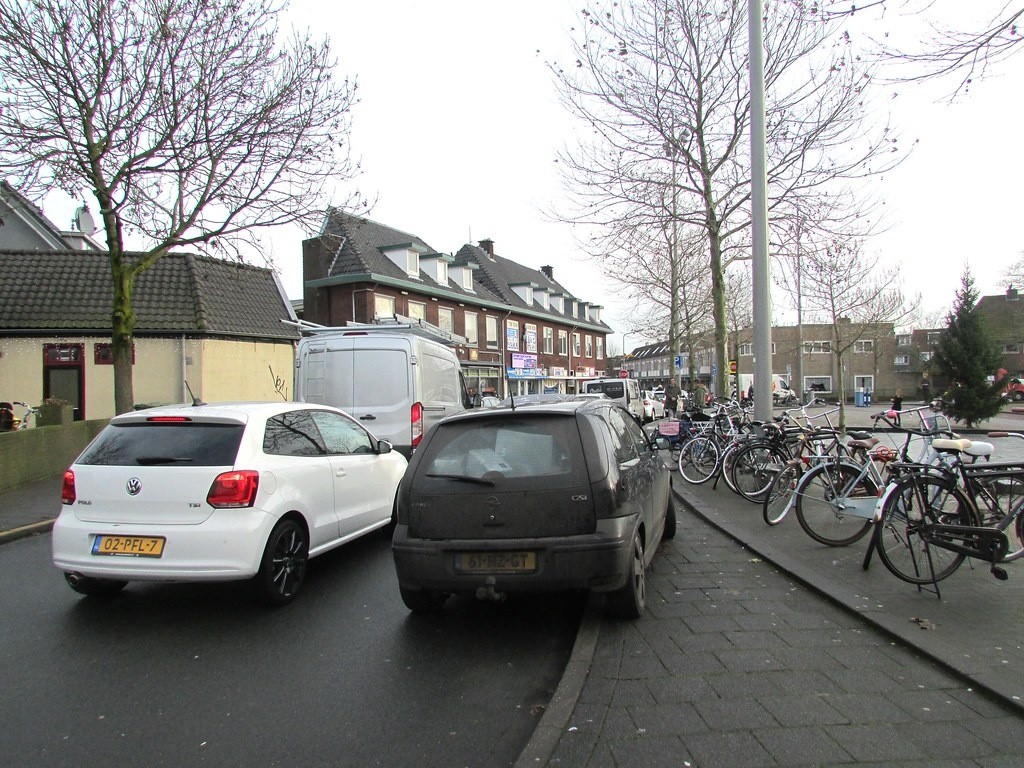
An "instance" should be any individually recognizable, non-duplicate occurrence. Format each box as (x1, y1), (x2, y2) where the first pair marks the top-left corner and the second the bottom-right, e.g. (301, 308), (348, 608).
(748, 373), (797, 407)
(640, 387), (690, 421)
(984, 375), (1024, 402)
(392, 395), (677, 619)
(52, 403), (410, 606)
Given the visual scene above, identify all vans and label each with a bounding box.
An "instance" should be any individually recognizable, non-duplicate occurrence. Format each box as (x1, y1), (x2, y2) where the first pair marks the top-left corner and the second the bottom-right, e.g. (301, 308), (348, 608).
(582, 378), (645, 426)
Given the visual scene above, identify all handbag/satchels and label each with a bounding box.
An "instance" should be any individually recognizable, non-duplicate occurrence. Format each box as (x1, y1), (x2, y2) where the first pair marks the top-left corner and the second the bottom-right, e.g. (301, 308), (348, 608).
(676, 395), (684, 411)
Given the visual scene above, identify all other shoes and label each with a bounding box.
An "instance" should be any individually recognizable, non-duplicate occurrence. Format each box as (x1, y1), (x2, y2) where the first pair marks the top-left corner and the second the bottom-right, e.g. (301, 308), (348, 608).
(893, 423), (901, 426)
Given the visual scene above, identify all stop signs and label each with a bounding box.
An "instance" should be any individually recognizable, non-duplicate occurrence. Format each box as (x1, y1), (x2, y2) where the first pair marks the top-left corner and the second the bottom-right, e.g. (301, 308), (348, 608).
(620, 371), (627, 378)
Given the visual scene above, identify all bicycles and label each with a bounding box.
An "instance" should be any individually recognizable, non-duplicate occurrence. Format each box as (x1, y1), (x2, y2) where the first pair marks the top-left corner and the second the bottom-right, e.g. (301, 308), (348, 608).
(859, 417), (1024, 572)
(872, 428), (1024, 598)
(651, 394), (941, 547)
(13, 398), (43, 430)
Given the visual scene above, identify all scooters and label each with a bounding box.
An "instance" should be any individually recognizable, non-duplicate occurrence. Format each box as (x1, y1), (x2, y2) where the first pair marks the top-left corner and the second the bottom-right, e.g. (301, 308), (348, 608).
(0, 402), (21, 433)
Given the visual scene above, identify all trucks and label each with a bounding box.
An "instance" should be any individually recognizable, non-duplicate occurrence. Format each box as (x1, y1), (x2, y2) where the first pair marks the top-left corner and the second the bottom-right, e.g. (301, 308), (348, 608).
(274, 314), (483, 463)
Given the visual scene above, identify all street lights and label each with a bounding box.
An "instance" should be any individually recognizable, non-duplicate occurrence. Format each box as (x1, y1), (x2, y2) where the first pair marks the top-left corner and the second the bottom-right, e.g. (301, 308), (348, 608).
(501, 310), (513, 399)
(621, 326), (644, 369)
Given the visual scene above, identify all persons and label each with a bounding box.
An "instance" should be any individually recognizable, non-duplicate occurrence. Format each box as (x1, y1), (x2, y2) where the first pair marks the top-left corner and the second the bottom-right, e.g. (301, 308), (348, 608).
(1010, 373), (1021, 384)
(664, 378), (681, 418)
(892, 389), (903, 426)
(748, 380), (754, 400)
(647, 384), (653, 391)
(655, 385), (663, 391)
(692, 379), (714, 408)
